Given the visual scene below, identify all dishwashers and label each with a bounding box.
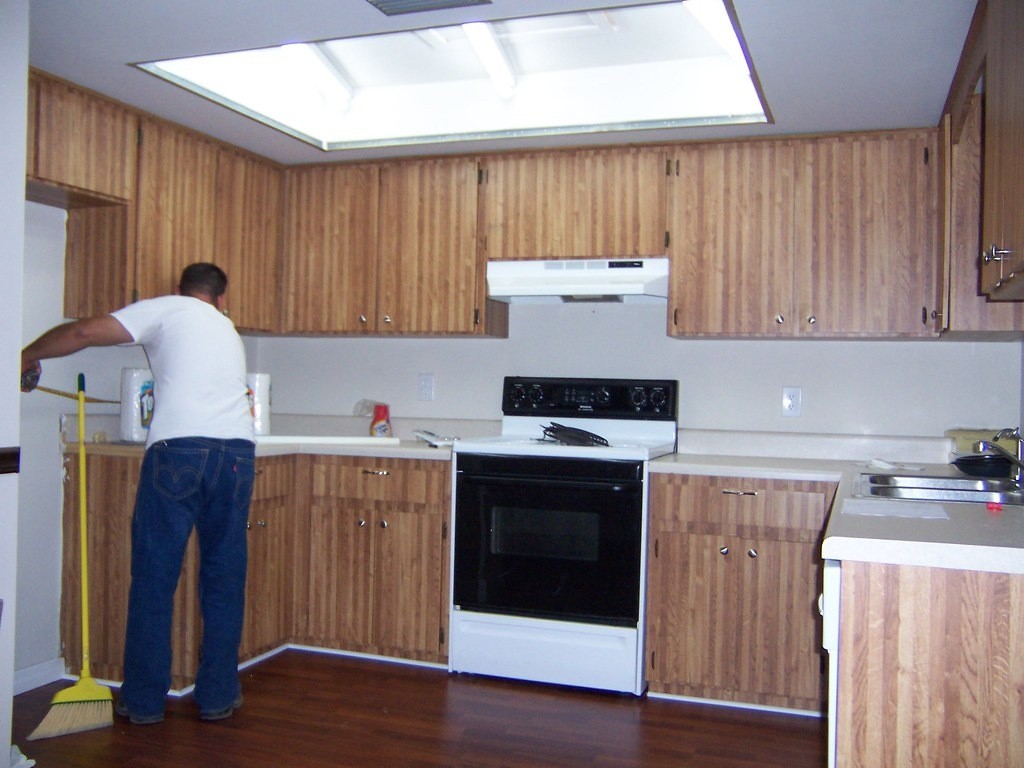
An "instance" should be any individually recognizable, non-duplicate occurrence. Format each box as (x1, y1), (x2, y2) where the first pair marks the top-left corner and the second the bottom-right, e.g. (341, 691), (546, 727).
(818, 560), (841, 768)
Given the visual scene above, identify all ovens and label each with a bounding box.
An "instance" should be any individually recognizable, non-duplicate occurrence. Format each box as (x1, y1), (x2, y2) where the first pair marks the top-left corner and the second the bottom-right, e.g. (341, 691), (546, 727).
(448, 451), (648, 696)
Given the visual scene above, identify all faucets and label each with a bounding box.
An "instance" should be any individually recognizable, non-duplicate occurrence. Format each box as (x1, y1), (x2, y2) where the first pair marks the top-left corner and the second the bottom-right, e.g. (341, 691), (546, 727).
(975, 441), (1024, 491)
(992, 428), (1021, 482)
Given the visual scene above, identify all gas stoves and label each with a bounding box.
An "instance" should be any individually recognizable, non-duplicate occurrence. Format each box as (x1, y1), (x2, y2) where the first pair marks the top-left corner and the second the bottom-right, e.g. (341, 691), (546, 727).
(453, 376), (680, 460)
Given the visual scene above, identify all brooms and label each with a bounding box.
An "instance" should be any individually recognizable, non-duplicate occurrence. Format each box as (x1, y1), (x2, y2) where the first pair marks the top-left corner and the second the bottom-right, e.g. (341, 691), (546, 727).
(22, 371), (116, 742)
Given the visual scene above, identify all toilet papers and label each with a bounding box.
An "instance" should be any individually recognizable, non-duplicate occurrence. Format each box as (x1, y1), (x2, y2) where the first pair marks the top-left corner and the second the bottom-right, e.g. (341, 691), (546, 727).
(119, 366), (157, 442)
(244, 373), (272, 436)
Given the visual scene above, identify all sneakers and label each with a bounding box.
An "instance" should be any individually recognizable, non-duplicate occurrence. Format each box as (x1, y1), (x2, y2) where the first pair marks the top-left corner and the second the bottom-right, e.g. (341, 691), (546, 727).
(115, 700), (164, 725)
(200, 692), (243, 720)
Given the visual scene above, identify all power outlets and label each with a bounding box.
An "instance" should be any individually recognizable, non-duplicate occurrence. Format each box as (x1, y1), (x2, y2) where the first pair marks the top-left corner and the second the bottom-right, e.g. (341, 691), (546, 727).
(780, 386), (802, 418)
(417, 372), (436, 402)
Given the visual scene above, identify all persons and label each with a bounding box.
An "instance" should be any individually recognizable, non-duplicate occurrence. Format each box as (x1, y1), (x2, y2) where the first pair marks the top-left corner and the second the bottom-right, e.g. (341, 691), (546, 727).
(20, 262), (255, 724)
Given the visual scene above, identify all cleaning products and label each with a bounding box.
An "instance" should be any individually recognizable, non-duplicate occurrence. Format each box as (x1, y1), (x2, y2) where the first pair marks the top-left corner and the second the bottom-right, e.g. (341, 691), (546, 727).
(369, 402), (393, 438)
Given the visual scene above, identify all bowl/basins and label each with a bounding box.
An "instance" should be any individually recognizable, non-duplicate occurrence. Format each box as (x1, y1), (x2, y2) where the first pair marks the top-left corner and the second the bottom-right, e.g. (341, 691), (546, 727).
(950, 455), (1013, 477)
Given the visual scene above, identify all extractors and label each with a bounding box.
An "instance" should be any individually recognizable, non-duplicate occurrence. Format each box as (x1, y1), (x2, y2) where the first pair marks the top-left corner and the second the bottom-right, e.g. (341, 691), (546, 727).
(486, 260), (669, 303)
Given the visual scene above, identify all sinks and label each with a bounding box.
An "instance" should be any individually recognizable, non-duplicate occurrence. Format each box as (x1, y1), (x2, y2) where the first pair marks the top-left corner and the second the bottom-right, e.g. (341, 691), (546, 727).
(850, 485), (1024, 507)
(858, 472), (1024, 492)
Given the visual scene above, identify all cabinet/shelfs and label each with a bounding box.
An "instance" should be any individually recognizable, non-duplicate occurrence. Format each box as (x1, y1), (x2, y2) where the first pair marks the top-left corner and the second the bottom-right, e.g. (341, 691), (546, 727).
(63, 110), (279, 338)
(297, 454), (452, 672)
(934, 106), (1024, 343)
(643, 473), (839, 718)
(484, 140), (667, 261)
(665, 127), (941, 342)
(976, 0), (1024, 303)
(26, 65), (138, 211)
(58, 452), (297, 698)
(279, 153), (509, 339)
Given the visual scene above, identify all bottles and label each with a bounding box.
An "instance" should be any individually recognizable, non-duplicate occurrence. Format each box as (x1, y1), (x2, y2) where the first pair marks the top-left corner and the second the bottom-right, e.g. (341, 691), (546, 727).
(370, 405), (391, 436)
